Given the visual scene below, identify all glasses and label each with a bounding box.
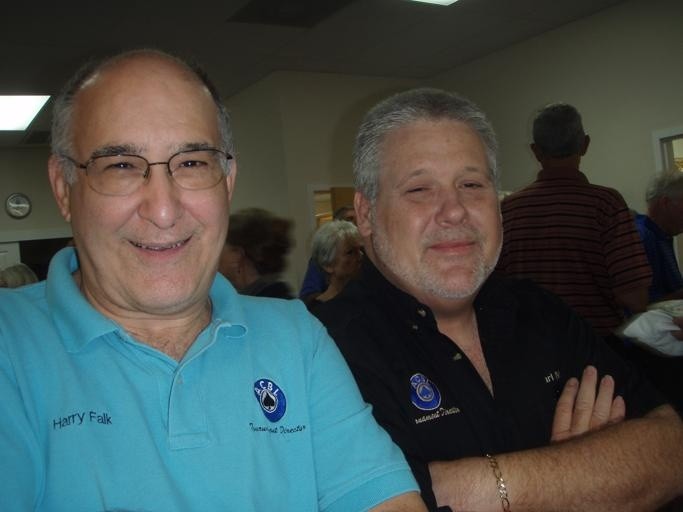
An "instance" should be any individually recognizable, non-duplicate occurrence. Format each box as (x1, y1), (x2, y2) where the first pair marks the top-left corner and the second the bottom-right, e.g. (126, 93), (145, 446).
(61, 147), (233, 197)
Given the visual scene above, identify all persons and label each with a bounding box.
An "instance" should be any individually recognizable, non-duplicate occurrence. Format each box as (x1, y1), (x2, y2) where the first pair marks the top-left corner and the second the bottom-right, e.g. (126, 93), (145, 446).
(0, 49), (425, 511)
(311, 87), (681, 512)
(298, 205), (372, 299)
(216, 208), (293, 298)
(304, 221), (362, 313)
(631, 169), (680, 297)
(500, 104), (650, 328)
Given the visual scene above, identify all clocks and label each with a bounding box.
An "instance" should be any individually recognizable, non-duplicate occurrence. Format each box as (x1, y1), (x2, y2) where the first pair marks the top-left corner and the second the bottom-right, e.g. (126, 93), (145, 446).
(4, 192), (32, 219)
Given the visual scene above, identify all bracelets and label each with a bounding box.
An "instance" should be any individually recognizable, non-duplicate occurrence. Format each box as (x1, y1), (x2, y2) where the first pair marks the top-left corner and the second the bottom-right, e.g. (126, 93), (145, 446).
(485, 452), (508, 511)
